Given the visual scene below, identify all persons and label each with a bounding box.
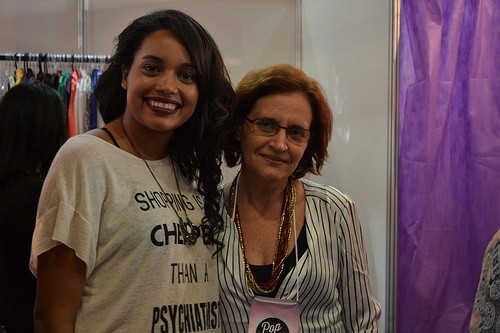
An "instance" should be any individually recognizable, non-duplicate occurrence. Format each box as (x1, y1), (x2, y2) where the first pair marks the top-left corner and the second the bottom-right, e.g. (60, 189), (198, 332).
(0, 10), (238, 333)
(213, 64), (381, 333)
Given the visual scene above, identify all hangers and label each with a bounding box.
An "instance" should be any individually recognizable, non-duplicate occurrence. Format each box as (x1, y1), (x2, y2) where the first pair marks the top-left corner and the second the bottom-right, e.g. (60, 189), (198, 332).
(1, 51), (110, 95)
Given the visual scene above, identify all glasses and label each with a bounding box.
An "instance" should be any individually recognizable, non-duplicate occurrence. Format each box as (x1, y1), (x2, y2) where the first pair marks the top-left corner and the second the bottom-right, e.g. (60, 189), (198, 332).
(244, 116), (315, 145)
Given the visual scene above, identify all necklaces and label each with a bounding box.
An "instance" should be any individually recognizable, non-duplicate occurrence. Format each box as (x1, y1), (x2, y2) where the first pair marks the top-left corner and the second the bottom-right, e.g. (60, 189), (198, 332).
(120, 116), (197, 246)
(228, 171), (298, 295)
